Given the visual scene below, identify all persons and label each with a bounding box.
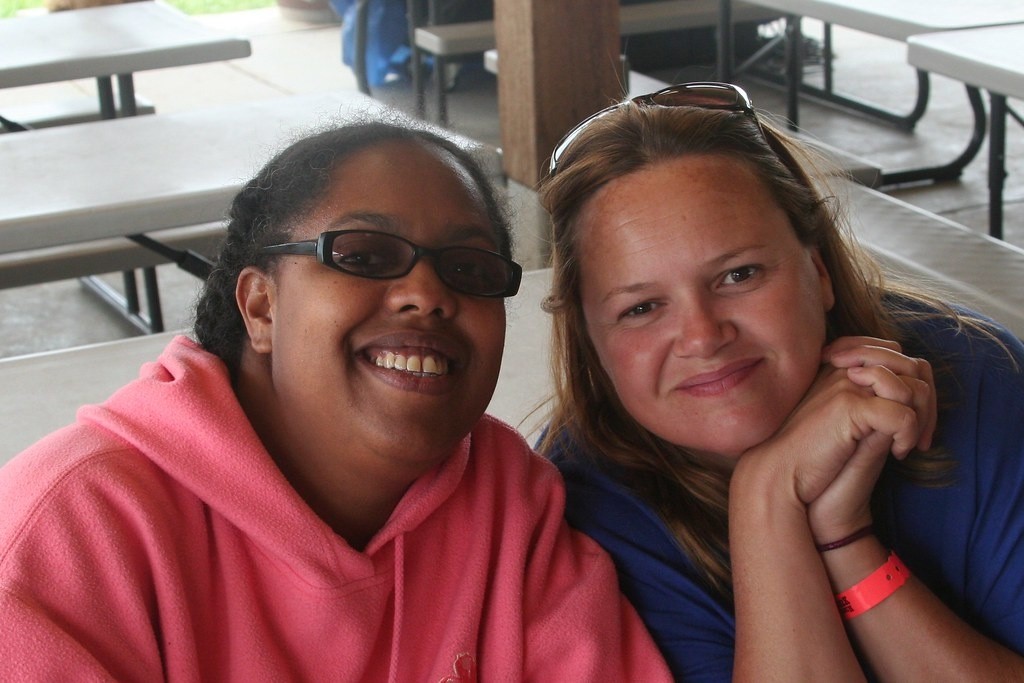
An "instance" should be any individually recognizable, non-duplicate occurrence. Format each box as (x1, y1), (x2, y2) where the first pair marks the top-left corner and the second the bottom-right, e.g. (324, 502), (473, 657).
(0, 120), (674, 683)
(533, 81), (1024, 683)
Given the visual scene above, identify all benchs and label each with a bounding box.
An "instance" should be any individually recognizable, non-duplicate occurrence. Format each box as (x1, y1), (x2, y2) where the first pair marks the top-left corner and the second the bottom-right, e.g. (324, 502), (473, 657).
(0, 264), (558, 465)
(0, 220), (229, 338)
(482, 48), (886, 197)
(810, 174), (1023, 342)
(0, 75), (156, 130)
(415, 0), (787, 123)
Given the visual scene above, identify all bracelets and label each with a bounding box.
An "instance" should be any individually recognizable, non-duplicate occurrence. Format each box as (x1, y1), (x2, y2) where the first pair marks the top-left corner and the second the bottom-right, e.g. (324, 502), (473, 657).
(815, 524), (876, 552)
(835, 551), (911, 620)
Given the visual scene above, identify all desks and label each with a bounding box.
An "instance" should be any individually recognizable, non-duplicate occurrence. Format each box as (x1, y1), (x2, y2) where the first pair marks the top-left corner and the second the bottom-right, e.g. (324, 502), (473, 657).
(748, 0), (1024, 183)
(0, 0), (253, 135)
(0, 81), (508, 282)
(907, 21), (1023, 240)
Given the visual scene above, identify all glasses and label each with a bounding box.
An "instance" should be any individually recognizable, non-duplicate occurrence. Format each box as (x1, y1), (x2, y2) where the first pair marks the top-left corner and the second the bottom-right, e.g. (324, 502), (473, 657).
(547, 82), (771, 183)
(256, 229), (522, 298)
